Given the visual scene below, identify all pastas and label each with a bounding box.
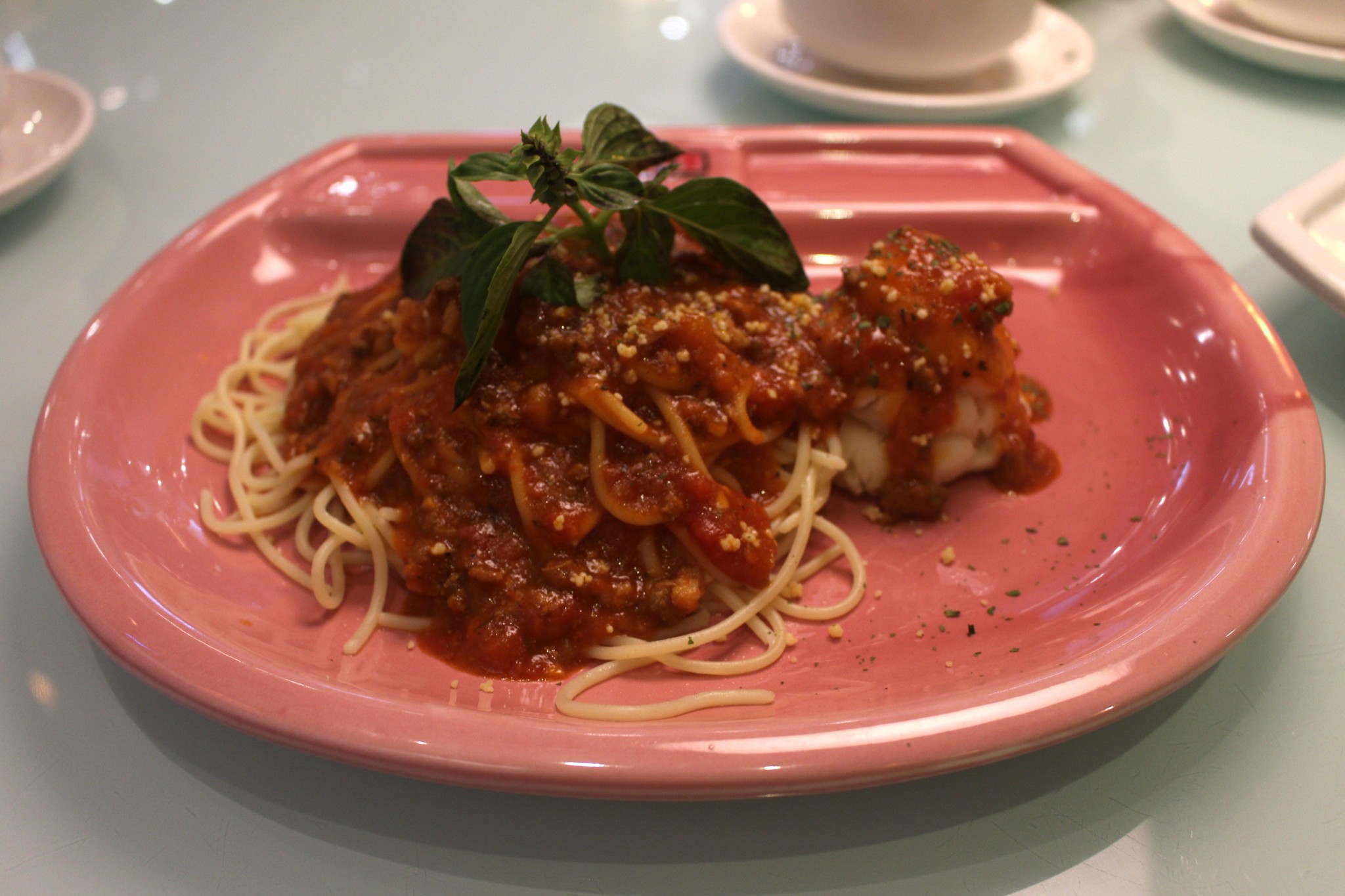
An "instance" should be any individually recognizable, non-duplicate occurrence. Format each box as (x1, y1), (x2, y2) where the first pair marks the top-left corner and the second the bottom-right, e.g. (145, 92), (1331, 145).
(192, 241), (867, 717)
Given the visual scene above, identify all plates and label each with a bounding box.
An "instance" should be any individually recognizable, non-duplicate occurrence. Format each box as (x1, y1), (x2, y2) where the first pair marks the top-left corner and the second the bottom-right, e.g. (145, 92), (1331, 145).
(0, 61), (96, 218)
(27, 116), (1330, 801)
(715, 0), (1095, 127)
(1250, 149), (1345, 310)
(1165, 0), (1345, 86)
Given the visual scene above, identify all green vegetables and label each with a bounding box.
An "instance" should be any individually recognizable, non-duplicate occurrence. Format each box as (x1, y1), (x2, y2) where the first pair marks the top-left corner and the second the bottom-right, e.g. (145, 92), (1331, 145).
(395, 95), (811, 413)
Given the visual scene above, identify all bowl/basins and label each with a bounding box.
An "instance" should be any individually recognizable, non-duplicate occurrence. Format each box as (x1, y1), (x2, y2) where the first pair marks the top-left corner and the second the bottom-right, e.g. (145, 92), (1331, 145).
(1230, 1), (1345, 46)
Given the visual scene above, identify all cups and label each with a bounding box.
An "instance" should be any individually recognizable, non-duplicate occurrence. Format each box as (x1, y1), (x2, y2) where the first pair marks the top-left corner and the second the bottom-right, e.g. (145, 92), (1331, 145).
(778, 0), (1043, 82)
